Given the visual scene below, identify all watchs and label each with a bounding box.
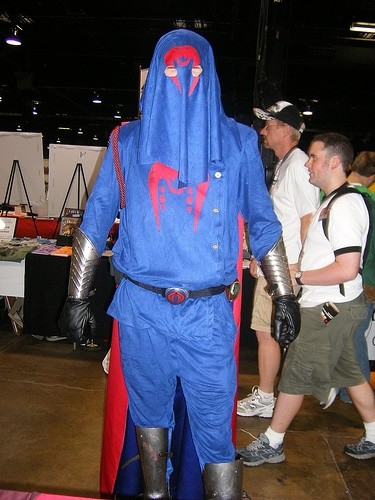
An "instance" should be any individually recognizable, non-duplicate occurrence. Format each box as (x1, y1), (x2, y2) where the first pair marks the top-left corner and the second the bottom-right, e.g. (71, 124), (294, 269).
(294, 271), (303, 286)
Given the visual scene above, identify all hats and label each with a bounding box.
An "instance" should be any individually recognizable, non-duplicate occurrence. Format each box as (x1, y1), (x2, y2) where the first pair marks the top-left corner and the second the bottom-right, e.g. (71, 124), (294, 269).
(252, 100), (306, 134)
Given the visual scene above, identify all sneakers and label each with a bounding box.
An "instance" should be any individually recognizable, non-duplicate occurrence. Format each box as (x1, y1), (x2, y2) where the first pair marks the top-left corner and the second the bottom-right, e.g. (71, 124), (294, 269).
(344, 437), (375, 459)
(235, 433), (286, 467)
(237, 385), (276, 418)
(320, 388), (337, 410)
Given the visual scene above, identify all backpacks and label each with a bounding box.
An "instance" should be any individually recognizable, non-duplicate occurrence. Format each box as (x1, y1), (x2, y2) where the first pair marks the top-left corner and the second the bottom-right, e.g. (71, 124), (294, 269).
(321, 185), (375, 287)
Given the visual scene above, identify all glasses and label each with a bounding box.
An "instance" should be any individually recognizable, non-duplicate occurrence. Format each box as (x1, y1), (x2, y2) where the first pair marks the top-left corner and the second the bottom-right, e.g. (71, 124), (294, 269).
(266, 125), (288, 131)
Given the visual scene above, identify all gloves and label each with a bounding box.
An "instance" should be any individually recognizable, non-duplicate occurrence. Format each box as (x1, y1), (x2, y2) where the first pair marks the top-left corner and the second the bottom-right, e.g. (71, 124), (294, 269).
(273, 296), (301, 347)
(59, 299), (99, 344)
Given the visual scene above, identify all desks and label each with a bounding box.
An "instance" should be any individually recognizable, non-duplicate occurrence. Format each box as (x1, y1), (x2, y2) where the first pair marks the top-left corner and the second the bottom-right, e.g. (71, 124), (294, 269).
(0, 240), (258, 352)
(0, 213), (119, 239)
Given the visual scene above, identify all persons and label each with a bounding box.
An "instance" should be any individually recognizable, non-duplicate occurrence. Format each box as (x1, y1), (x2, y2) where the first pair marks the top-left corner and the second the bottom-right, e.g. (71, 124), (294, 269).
(66, 29), (300, 500)
(232, 100), (374, 464)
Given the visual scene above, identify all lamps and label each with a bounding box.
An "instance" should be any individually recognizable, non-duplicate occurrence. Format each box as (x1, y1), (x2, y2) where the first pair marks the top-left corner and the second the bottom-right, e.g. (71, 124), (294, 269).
(0, 0), (318, 143)
